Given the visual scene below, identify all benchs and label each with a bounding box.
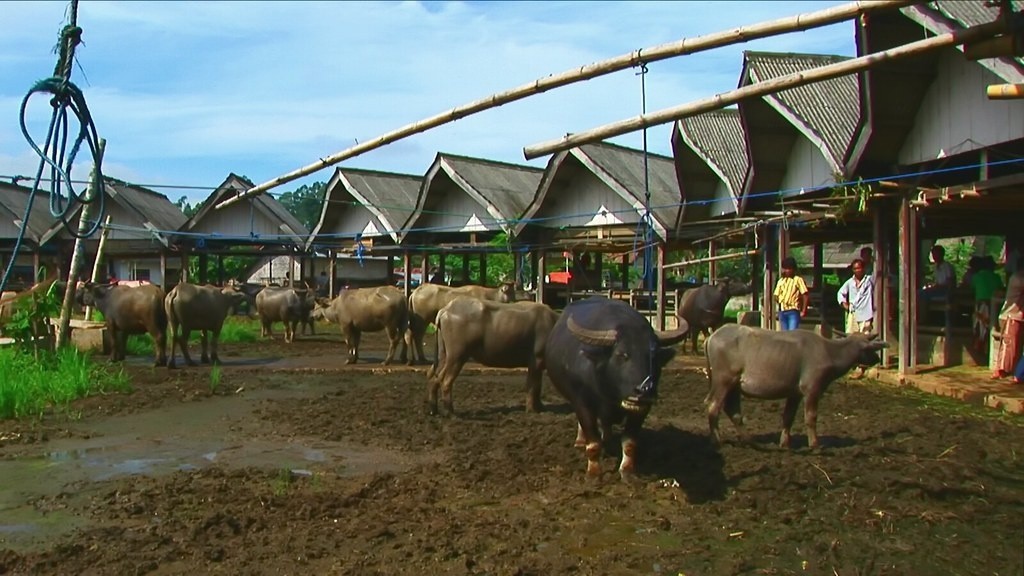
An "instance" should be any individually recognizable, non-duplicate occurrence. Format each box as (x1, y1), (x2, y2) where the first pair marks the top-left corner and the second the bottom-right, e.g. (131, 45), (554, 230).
(915, 325), (983, 366)
(45, 316), (108, 355)
(796, 315), (846, 340)
(636, 309), (677, 330)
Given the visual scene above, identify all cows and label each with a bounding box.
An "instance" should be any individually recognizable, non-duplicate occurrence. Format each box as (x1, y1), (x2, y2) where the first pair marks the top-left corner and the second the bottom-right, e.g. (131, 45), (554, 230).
(677, 276), (752, 356)
(164, 283), (245, 366)
(542, 297), (690, 479)
(409, 282), (516, 364)
(426, 296), (560, 418)
(703, 323), (891, 449)
(256, 280), (320, 344)
(312, 286), (413, 364)
(76, 278), (166, 364)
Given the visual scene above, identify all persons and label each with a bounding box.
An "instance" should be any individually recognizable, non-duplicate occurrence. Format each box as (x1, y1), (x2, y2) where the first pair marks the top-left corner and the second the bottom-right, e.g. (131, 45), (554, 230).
(339, 280), (351, 296)
(932, 246), (956, 290)
(773, 257), (808, 331)
(0, 267), (24, 292)
(107, 272), (120, 285)
(961, 256), (1024, 384)
(430, 269), (441, 284)
(316, 271), (329, 297)
(285, 272), (290, 281)
(837, 251), (877, 379)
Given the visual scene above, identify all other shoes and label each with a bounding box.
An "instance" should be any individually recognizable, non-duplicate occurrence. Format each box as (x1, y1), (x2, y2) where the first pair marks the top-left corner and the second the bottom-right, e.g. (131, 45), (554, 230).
(851, 371), (863, 379)
(990, 371), (1004, 379)
(1009, 377), (1019, 383)
